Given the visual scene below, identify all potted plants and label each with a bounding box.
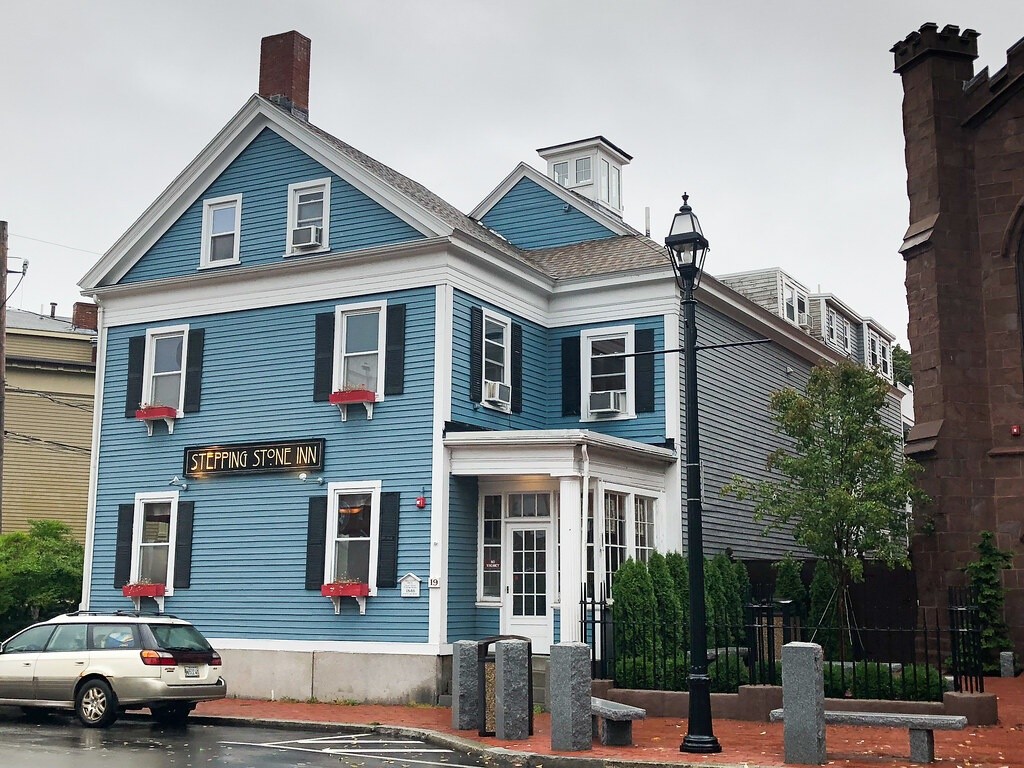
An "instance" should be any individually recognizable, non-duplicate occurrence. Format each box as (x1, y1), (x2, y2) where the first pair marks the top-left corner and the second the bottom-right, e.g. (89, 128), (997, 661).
(321, 569), (369, 597)
(122, 575), (166, 597)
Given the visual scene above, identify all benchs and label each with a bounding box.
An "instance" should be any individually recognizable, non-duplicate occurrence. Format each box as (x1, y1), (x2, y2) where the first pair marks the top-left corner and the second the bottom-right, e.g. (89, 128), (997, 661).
(768, 707), (968, 762)
(591, 696), (645, 745)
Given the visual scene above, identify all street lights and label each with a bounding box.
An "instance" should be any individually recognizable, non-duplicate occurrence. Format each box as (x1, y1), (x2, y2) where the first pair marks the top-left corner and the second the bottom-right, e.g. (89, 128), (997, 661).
(664, 192), (723, 754)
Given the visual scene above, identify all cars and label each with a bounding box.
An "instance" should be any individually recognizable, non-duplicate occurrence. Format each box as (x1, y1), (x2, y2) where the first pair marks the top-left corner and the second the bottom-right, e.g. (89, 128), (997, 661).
(0, 609), (226, 730)
